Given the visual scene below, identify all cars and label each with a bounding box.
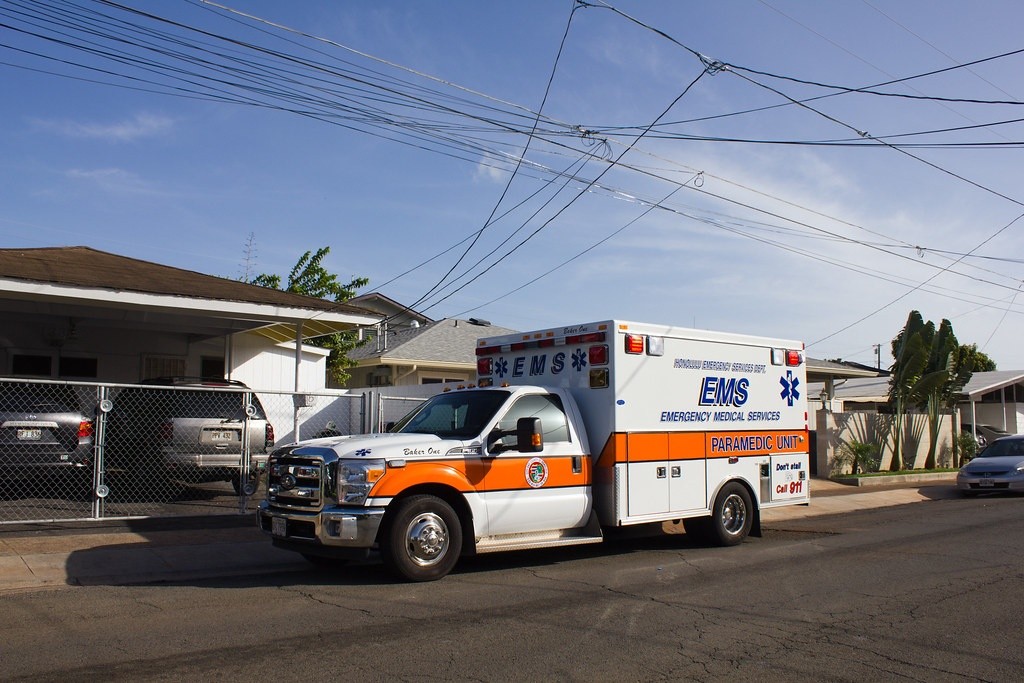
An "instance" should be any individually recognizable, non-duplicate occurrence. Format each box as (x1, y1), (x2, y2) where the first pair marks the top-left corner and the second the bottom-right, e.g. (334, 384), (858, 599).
(960, 421), (1014, 443)
(955, 433), (1023, 498)
(958, 424), (988, 457)
(0, 374), (93, 485)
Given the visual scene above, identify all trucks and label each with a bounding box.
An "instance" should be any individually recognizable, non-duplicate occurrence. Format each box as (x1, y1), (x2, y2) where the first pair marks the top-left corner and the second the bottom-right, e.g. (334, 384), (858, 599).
(249, 320), (812, 579)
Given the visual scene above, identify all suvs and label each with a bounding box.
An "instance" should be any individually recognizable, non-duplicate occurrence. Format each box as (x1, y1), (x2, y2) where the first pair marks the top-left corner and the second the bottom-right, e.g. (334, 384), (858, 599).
(105, 374), (278, 501)
